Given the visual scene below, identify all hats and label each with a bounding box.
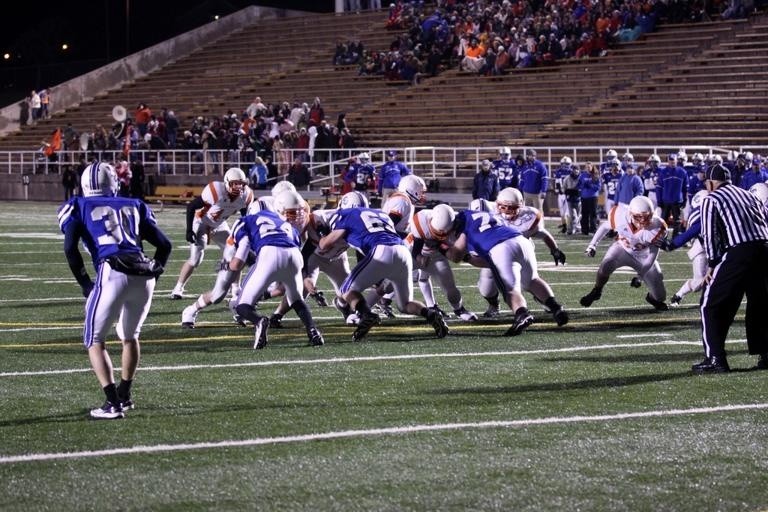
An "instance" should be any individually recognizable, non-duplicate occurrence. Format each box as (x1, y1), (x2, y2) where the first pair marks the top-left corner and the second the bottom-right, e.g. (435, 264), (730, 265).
(388, 151), (396, 156)
(697, 164), (731, 182)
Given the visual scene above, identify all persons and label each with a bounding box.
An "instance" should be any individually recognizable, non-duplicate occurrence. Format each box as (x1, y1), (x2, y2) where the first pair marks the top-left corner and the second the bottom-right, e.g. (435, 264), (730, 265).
(169, 147), (768, 350)
(332, 1), (768, 87)
(29, 89), (51, 126)
(56, 163), (173, 421)
(39, 95), (356, 204)
(688, 163), (766, 374)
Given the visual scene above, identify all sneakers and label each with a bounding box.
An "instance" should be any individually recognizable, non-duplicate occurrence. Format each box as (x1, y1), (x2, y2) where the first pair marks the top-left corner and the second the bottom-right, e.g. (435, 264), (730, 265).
(171, 286), (183, 299)
(505, 310), (533, 335)
(645, 291), (668, 311)
(89, 399), (134, 419)
(580, 288), (601, 307)
(553, 305), (568, 326)
(427, 305), (477, 338)
(692, 355), (730, 373)
(180, 307), (195, 327)
(671, 294), (682, 307)
(233, 291), (327, 349)
(332, 296), (395, 342)
(482, 304), (499, 318)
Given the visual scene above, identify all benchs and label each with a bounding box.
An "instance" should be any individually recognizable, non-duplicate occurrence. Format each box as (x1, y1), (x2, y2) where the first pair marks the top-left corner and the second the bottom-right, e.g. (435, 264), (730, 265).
(0, 10), (767, 177)
(144, 185), (209, 210)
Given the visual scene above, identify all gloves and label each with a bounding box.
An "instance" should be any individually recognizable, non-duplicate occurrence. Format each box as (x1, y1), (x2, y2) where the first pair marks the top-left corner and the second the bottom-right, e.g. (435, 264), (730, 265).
(584, 245), (596, 257)
(215, 258), (230, 272)
(655, 236), (673, 252)
(186, 231), (197, 243)
(551, 248), (566, 265)
(631, 277), (641, 288)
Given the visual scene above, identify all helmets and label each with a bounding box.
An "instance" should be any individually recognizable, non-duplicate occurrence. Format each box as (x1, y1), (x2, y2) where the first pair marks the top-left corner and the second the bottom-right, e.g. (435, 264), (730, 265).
(647, 154), (660, 168)
(482, 147), (536, 166)
(430, 204), (454, 241)
(398, 174), (426, 205)
(358, 152), (369, 164)
(80, 161), (120, 198)
(338, 191), (368, 210)
(250, 180), (305, 221)
(605, 150), (617, 160)
(560, 155), (580, 176)
(496, 187), (522, 220)
(667, 149), (754, 171)
(691, 189), (709, 208)
(622, 153), (633, 161)
(748, 182), (768, 206)
(629, 195), (655, 230)
(224, 167), (248, 196)
